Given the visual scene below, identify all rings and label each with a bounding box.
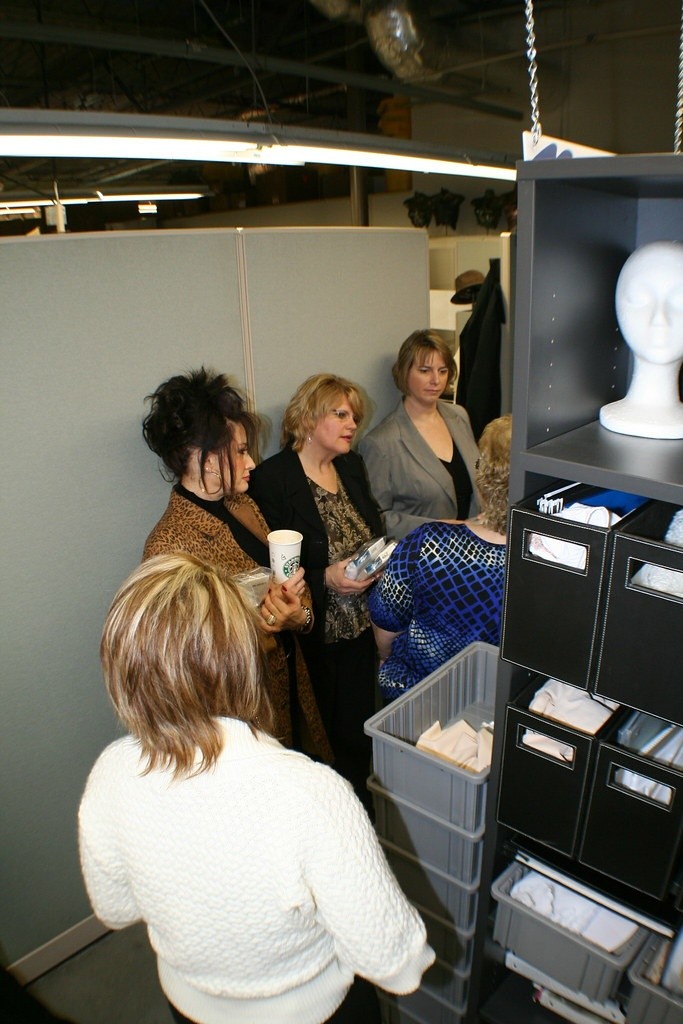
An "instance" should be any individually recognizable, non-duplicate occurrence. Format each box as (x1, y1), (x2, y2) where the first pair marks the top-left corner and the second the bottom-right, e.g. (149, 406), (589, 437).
(265, 614), (276, 626)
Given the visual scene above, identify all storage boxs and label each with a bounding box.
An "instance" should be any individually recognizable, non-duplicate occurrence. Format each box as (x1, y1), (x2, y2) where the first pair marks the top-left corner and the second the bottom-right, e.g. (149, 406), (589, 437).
(491, 861), (649, 1001)
(495, 675), (683, 930)
(362, 641), (496, 1024)
(626, 933), (683, 1024)
(500, 482), (683, 726)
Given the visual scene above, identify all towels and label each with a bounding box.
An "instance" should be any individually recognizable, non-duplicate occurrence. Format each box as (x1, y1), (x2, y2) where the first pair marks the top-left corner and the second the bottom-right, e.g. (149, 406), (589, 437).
(524, 489), (649, 568)
(630, 508), (682, 597)
(521, 677), (620, 762)
(511, 870), (640, 952)
(413, 719), (496, 772)
(612, 710), (682, 806)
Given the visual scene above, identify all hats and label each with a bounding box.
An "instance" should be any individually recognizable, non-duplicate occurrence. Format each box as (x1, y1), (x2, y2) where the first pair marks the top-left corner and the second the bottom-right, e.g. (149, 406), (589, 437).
(450, 270), (486, 305)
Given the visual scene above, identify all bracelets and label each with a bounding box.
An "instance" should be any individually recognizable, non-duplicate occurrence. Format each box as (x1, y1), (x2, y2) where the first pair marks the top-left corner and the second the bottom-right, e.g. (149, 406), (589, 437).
(377, 653), (389, 662)
(296, 604), (312, 633)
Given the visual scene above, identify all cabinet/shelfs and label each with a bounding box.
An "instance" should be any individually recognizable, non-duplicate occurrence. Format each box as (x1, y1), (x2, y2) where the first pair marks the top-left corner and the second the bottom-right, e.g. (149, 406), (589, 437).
(464, 154), (683, 1024)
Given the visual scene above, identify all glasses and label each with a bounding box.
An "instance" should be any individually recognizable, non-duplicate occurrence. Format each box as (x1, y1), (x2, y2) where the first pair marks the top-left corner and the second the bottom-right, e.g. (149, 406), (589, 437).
(330, 409), (359, 426)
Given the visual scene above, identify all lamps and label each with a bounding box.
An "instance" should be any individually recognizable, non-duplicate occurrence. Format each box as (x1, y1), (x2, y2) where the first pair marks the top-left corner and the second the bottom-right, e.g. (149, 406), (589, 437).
(0, 109), (521, 208)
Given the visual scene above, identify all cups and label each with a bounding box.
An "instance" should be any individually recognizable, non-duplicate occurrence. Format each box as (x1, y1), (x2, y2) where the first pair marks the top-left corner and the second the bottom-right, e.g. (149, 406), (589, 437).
(267, 529), (303, 585)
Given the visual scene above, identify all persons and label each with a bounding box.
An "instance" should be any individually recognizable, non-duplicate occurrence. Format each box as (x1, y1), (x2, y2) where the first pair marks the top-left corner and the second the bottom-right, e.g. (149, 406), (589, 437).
(77, 551), (437, 1024)
(243, 374), (394, 822)
(364, 410), (513, 711)
(140, 364), (339, 771)
(599, 239), (683, 439)
(354, 327), (483, 543)
